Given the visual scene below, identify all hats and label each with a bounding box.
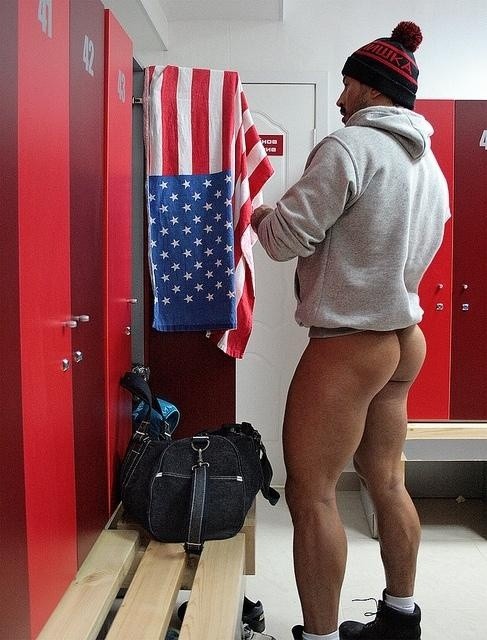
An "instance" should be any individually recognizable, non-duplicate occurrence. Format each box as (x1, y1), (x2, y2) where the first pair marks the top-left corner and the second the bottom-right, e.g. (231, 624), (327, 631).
(341, 19), (422, 111)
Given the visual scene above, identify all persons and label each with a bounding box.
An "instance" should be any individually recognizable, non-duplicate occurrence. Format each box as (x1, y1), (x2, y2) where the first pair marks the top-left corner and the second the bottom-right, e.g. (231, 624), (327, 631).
(250, 22), (455, 639)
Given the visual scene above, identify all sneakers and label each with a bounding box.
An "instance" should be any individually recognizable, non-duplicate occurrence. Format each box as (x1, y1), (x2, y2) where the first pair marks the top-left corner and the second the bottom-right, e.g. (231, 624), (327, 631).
(168, 621), (275, 640)
(177, 595), (266, 633)
(338, 592), (421, 639)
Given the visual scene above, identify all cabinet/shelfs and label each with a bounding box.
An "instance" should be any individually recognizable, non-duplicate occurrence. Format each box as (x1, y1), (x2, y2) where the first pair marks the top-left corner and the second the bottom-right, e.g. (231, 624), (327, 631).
(406, 99), (487, 421)
(0, 0), (237, 640)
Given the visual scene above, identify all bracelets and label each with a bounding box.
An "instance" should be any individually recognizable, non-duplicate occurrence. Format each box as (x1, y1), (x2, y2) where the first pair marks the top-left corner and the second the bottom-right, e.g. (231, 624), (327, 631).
(254, 211), (271, 228)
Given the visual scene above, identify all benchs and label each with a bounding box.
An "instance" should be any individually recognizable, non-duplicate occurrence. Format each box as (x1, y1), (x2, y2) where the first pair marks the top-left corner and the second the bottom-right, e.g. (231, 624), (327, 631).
(359, 421), (487, 539)
(35, 500), (255, 640)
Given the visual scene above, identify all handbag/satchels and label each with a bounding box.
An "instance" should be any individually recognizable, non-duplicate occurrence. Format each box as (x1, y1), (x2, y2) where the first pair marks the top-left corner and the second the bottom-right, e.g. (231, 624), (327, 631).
(118, 371), (280, 558)
